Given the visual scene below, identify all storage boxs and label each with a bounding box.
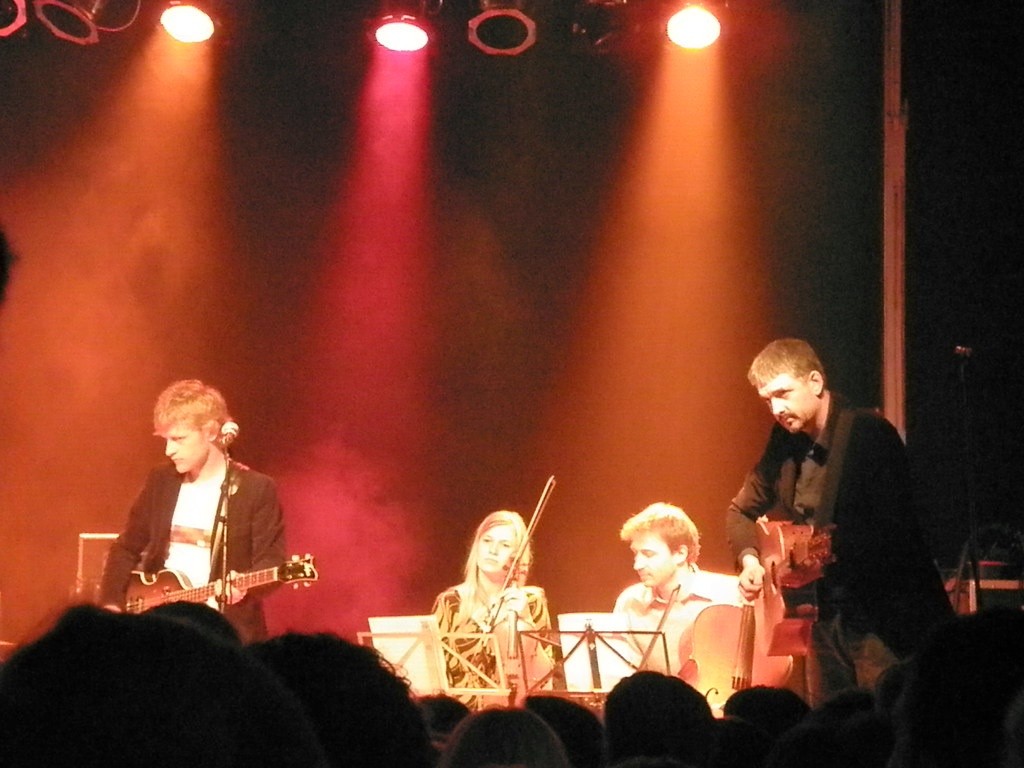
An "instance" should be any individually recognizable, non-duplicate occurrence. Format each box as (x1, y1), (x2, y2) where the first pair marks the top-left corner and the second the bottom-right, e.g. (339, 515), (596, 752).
(978, 578), (1021, 609)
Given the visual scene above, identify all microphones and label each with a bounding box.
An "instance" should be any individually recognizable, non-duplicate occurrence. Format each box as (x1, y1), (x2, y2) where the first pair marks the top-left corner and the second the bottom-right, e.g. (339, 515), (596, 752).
(222, 422), (239, 448)
(505, 581), (520, 659)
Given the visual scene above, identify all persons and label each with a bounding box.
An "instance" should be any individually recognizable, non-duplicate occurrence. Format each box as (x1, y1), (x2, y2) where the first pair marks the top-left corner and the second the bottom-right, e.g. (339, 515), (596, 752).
(97, 381), (284, 646)
(726, 337), (958, 708)
(434, 510), (553, 712)
(615, 500), (747, 680)
(1, 601), (1024, 768)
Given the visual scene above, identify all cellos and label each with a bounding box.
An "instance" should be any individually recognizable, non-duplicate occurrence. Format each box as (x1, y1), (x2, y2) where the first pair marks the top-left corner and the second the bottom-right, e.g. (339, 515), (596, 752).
(676, 594), (804, 718)
(479, 553), (539, 710)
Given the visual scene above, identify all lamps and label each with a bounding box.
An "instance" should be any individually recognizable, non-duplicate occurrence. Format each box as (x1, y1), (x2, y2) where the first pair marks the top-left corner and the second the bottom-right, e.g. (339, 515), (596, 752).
(663, 0), (721, 49)
(156, 0), (215, 43)
(373, 0), (435, 51)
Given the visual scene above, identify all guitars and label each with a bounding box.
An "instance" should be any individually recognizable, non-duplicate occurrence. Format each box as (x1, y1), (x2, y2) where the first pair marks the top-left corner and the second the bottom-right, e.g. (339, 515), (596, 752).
(123, 551), (320, 617)
(754, 509), (832, 657)
